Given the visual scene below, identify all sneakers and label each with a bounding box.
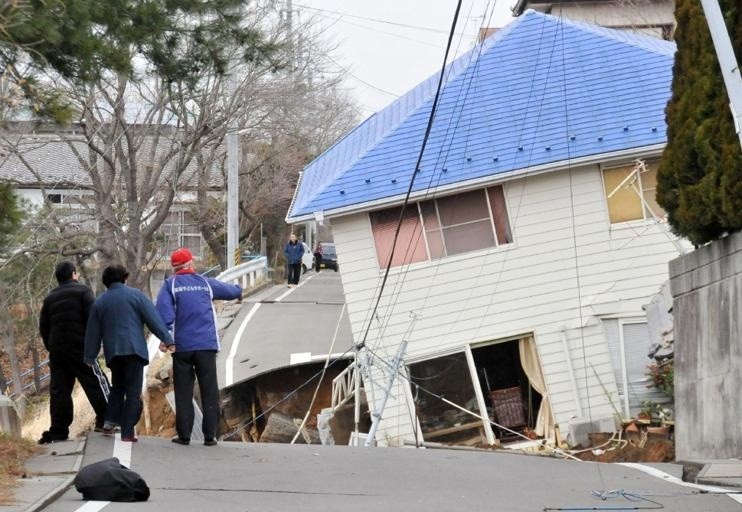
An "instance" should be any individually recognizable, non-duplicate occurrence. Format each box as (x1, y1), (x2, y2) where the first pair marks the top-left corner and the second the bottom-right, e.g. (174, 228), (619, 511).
(288, 283), (292, 287)
(205, 439), (217, 445)
(172, 435), (189, 445)
(122, 437), (138, 442)
(94, 421), (121, 433)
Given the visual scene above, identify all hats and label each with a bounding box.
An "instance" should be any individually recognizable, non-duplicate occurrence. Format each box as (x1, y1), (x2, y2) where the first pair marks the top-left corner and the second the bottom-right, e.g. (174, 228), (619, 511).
(102, 265), (128, 283)
(171, 248), (192, 267)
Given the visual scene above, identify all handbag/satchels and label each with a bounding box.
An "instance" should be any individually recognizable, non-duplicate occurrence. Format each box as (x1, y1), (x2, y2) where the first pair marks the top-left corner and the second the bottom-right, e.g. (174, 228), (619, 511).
(75, 457), (149, 502)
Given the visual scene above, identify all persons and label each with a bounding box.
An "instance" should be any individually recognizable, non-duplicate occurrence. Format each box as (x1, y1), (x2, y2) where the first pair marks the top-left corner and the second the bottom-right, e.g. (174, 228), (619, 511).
(38, 261), (112, 445)
(283, 233), (305, 288)
(154, 247), (243, 445)
(84, 264), (176, 443)
(314, 249), (324, 272)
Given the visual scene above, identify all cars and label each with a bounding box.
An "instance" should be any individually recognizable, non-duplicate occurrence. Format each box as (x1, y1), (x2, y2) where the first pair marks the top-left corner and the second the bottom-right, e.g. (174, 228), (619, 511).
(300, 242), (316, 275)
(314, 243), (339, 272)
